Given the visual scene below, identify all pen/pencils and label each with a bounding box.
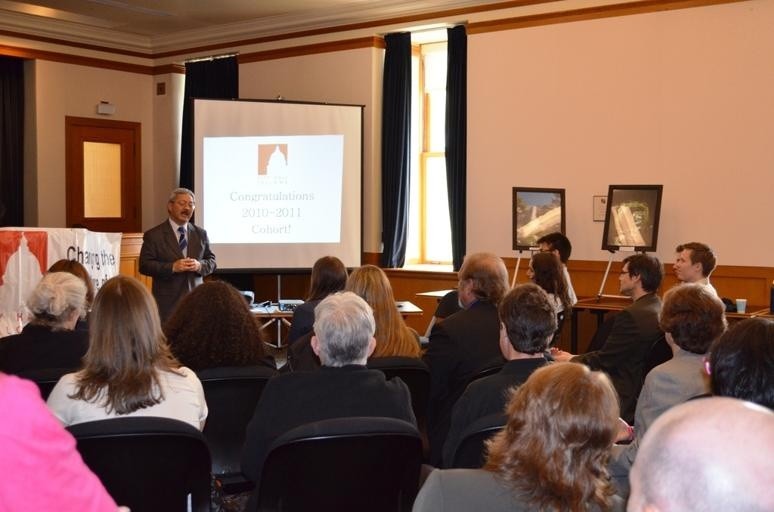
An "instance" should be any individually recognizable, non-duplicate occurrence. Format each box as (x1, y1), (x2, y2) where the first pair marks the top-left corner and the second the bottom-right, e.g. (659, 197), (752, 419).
(398, 305), (403, 307)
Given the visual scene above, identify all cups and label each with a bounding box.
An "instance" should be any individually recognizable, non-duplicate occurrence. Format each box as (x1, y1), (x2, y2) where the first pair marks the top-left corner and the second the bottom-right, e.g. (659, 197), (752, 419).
(737, 299), (747, 314)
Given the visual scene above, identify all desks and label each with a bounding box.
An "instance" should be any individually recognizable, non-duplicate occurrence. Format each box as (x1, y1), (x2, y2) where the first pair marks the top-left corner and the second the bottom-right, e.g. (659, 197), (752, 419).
(572, 296), (767, 365)
(120, 232), (153, 295)
(416, 286), (455, 337)
(252, 301), (423, 348)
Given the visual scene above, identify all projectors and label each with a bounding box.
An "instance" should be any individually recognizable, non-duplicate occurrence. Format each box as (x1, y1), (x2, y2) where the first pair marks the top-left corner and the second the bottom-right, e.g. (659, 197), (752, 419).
(279, 299), (306, 312)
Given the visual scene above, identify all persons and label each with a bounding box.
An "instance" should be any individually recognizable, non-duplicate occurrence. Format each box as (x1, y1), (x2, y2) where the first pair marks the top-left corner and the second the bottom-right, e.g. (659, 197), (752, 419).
(527, 251), (573, 332)
(411, 364), (628, 511)
(689, 318), (772, 416)
(163, 279), (279, 373)
(623, 394), (773, 511)
(674, 242), (719, 297)
(0, 272), (88, 396)
(551, 256), (665, 420)
(345, 265), (420, 360)
(440, 285), (560, 466)
(538, 235), (576, 308)
(0, 370), (132, 512)
(636, 285), (727, 441)
(48, 259), (92, 314)
(249, 292), (419, 504)
(138, 188), (217, 323)
(289, 256), (347, 342)
(47, 277), (208, 431)
(426, 253), (510, 436)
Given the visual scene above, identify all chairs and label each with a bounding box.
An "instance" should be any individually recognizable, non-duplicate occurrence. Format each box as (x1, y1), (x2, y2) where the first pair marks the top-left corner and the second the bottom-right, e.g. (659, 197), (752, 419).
(365, 357), (428, 408)
(251, 415), (426, 512)
(64, 416), (215, 512)
(178, 362), (285, 443)
(20, 367), (79, 399)
(445, 362), (507, 405)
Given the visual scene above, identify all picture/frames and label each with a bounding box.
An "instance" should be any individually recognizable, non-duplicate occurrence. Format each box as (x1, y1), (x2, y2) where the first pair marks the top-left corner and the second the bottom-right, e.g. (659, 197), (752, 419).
(511, 186), (565, 253)
(601, 185), (662, 253)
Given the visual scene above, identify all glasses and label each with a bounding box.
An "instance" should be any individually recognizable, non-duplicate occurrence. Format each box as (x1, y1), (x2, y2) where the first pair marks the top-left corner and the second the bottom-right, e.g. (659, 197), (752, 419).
(539, 247), (554, 254)
(172, 199), (195, 208)
(619, 269), (635, 276)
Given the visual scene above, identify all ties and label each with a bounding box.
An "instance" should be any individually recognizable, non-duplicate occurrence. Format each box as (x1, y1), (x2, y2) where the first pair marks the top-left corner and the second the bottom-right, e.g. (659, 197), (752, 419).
(178, 226), (188, 251)
